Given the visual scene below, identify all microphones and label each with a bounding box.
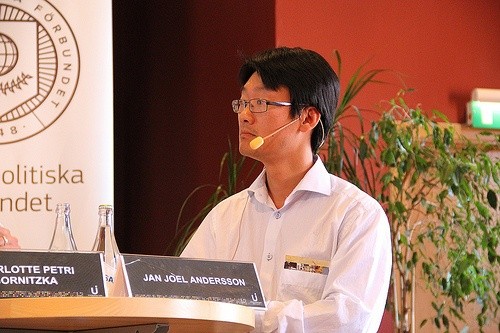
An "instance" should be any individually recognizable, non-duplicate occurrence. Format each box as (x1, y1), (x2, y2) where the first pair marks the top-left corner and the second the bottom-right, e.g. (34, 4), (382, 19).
(249, 117), (301, 150)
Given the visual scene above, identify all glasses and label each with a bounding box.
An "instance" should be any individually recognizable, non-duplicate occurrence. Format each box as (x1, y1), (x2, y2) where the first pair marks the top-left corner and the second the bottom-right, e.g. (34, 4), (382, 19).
(232, 98), (309, 113)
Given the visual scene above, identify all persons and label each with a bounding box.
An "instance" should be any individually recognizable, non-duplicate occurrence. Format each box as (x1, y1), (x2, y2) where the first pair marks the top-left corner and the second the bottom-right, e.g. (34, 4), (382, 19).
(179, 47), (392, 333)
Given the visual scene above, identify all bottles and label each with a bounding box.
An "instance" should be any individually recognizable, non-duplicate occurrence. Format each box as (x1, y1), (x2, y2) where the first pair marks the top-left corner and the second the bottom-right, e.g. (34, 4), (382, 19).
(91, 204), (121, 297)
(49, 203), (78, 253)
